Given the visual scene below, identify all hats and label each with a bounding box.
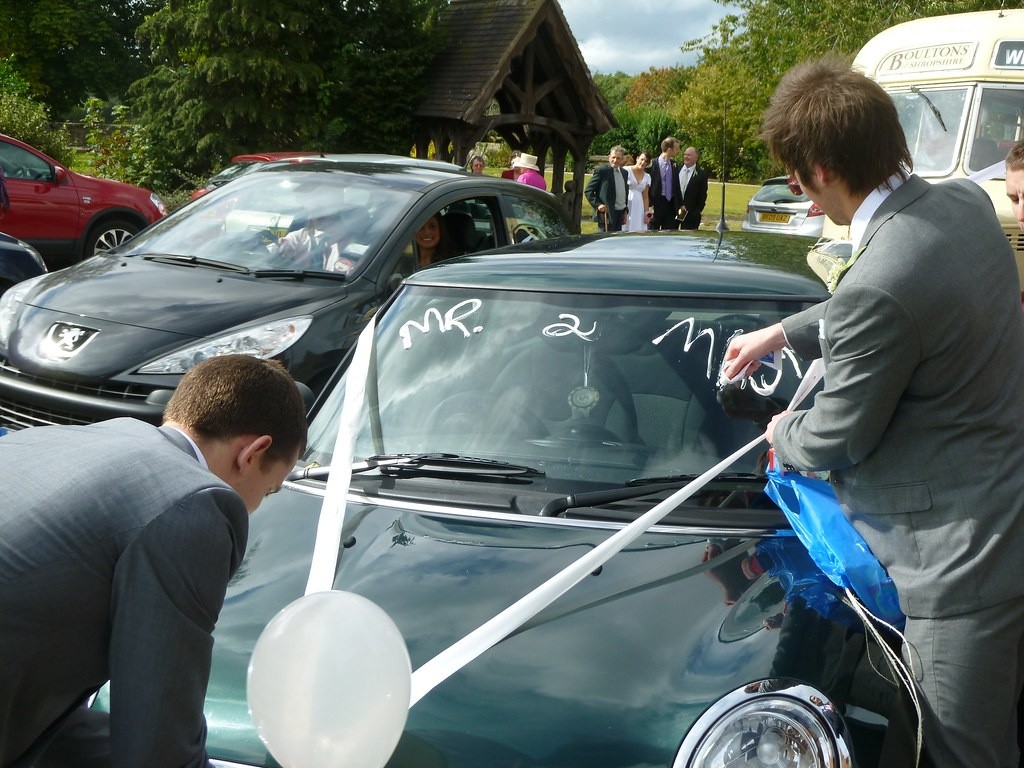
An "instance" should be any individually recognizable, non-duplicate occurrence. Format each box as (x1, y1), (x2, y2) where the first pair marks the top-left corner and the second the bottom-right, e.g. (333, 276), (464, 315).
(514, 153), (539, 171)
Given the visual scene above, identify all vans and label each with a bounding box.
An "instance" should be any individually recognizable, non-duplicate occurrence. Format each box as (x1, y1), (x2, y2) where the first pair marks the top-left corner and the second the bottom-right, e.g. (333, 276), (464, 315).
(818, 8), (1024, 292)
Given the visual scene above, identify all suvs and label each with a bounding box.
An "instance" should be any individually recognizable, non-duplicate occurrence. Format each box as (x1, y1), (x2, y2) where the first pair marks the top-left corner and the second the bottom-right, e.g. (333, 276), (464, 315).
(0, 135), (168, 268)
(741, 176), (828, 237)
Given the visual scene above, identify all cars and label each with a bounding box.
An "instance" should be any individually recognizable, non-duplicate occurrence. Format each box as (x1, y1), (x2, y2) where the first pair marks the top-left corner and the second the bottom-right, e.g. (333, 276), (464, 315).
(64, 227), (915, 768)
(186, 150), (543, 260)
(1, 162), (580, 430)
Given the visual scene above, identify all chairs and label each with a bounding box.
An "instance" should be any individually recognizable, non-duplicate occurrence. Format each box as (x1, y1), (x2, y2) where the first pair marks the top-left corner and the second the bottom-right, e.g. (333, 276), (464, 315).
(439, 212), (491, 252)
(504, 311), (816, 461)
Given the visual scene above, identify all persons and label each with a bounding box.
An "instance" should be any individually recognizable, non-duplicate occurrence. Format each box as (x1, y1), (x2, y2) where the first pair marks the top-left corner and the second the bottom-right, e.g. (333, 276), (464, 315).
(0, 355), (308, 768)
(264, 185), (350, 271)
(470, 155), (485, 175)
(1005, 140), (1024, 232)
(721, 55), (1024, 767)
(500, 149), (547, 191)
(404, 210), (468, 275)
(584, 136), (708, 233)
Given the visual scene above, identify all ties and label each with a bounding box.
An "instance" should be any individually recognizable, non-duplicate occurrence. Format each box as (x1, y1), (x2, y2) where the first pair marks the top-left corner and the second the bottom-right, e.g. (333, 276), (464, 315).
(681, 168), (689, 200)
(665, 159), (672, 202)
